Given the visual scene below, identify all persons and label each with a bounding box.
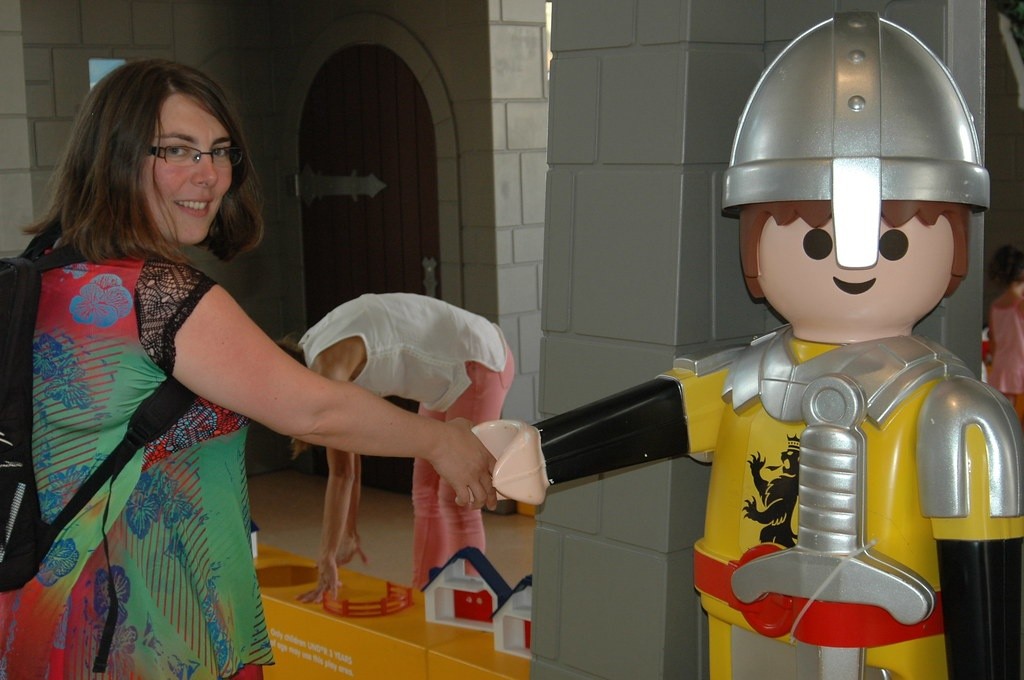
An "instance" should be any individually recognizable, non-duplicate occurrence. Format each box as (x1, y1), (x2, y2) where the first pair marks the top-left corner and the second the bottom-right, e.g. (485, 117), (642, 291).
(987, 245), (1024, 433)
(467, 13), (1024, 680)
(274, 293), (515, 606)
(0, 60), (497, 680)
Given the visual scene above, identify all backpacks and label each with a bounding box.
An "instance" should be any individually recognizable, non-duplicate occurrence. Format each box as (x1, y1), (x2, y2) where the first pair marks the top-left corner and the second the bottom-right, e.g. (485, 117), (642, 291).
(0, 227), (198, 589)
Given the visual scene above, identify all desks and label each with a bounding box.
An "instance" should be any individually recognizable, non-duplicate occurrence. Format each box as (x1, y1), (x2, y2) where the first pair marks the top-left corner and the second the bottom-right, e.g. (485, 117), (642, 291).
(254, 544), (533, 680)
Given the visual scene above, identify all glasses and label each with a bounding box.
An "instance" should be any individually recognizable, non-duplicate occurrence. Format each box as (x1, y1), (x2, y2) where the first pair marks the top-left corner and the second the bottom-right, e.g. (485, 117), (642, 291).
(143, 142), (244, 168)
